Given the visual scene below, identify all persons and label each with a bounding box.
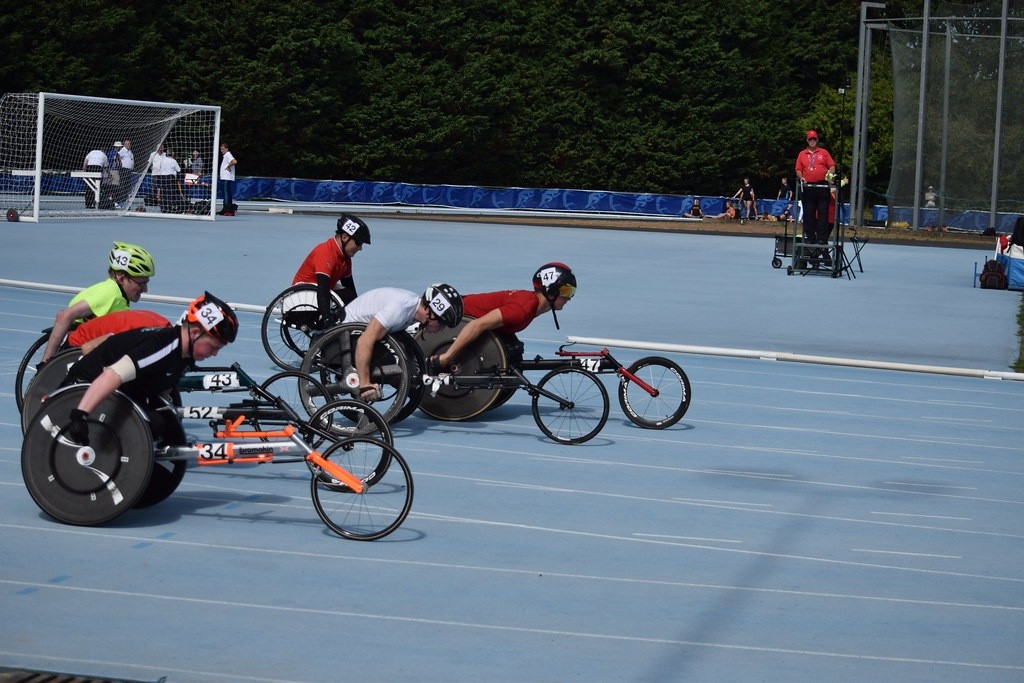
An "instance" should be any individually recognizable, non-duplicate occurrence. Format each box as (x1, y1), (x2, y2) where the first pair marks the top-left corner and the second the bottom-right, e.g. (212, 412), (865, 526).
(425, 262), (577, 371)
(83, 148), (112, 209)
(795, 130), (835, 244)
(59, 291), (240, 446)
(36, 241), (156, 371)
(734, 177), (758, 221)
(722, 201), (735, 219)
(149, 144), (180, 212)
(344, 283), (464, 401)
(192, 151), (203, 173)
(293, 213), (372, 313)
(220, 144), (237, 215)
(815, 188), (836, 266)
(777, 177), (792, 201)
(691, 199), (703, 216)
(759, 204), (793, 223)
(108, 141), (124, 185)
(925, 185), (936, 208)
(117, 140), (135, 187)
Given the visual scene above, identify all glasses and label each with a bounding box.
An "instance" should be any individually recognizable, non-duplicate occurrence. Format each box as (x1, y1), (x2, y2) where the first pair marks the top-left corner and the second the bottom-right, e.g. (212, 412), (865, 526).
(353, 239), (363, 246)
(128, 279), (147, 288)
(809, 138), (818, 141)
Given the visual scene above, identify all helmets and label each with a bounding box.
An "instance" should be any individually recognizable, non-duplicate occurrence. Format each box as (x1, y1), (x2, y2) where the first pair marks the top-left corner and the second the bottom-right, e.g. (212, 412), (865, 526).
(187, 290), (238, 342)
(106, 241), (154, 277)
(336, 213), (372, 244)
(533, 262), (577, 297)
(422, 283), (465, 327)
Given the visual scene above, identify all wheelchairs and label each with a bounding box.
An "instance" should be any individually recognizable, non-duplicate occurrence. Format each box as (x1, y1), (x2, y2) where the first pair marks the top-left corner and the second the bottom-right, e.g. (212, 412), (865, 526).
(20, 382), (414, 541)
(297, 321), (610, 445)
(261, 281), (691, 430)
(14, 321), (395, 493)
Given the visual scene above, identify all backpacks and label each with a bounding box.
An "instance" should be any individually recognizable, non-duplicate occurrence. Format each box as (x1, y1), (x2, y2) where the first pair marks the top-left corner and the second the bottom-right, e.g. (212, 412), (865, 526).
(980, 260), (1008, 290)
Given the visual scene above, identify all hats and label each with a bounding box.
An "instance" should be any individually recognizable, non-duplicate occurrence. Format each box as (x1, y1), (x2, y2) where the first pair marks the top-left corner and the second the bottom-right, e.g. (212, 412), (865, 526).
(112, 142), (124, 147)
(807, 130), (817, 139)
(158, 145), (164, 150)
(1000, 235), (1008, 255)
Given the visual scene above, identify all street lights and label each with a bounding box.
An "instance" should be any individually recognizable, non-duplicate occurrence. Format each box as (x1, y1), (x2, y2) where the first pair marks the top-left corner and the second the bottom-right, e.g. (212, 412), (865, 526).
(838, 77), (851, 223)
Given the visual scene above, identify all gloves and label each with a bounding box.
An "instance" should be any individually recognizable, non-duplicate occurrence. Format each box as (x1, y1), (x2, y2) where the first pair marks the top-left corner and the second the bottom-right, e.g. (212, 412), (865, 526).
(426, 355), (444, 377)
(317, 307), (332, 330)
(68, 409), (90, 447)
